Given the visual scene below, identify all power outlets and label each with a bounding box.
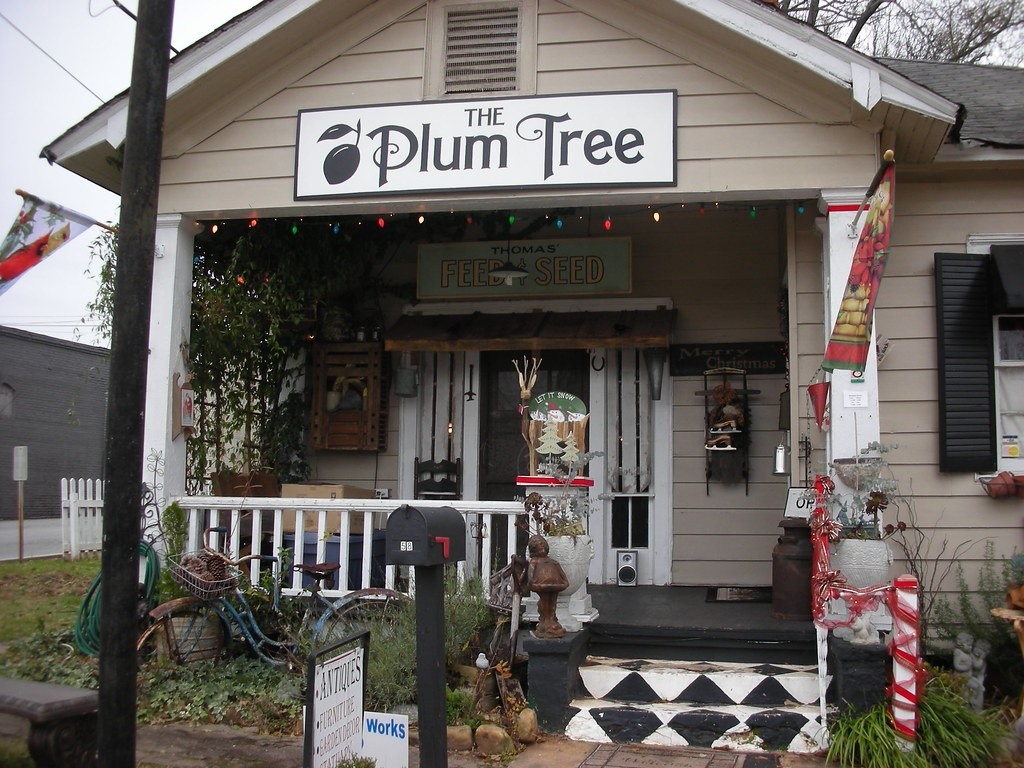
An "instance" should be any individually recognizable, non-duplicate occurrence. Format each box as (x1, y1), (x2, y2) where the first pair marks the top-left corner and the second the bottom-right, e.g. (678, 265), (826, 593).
(374, 488), (389, 498)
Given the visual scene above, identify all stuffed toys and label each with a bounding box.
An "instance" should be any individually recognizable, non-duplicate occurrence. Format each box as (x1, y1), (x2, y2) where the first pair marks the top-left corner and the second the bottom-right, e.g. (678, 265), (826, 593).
(707, 382), (744, 427)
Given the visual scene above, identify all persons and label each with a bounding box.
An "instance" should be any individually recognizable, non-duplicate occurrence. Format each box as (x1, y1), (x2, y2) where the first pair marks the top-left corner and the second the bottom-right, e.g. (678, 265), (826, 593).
(952, 632), (991, 712)
(526, 534), (570, 639)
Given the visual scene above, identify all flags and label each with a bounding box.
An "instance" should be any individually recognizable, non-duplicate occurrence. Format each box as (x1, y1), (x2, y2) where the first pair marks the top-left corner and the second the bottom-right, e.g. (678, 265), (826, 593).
(0, 194), (97, 291)
(821, 156), (897, 373)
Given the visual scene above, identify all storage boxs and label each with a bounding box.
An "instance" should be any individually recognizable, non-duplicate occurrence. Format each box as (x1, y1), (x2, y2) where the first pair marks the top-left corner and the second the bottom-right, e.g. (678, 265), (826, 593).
(281, 482), (376, 534)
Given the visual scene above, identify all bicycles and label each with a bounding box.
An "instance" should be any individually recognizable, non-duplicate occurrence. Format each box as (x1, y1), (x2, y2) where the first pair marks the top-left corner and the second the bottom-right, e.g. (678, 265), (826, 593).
(134, 526), (415, 669)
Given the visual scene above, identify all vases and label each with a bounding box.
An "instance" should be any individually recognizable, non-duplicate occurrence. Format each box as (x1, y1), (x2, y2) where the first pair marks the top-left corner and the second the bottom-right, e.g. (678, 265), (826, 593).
(525, 534), (595, 633)
(828, 538), (893, 630)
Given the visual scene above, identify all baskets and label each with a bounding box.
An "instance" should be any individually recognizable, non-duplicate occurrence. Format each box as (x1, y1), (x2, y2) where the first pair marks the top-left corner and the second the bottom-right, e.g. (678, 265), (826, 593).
(168, 550), (244, 601)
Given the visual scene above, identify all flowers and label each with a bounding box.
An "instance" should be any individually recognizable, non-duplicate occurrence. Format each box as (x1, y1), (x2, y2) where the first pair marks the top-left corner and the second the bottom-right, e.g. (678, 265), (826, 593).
(513, 451), (616, 545)
(835, 491), (906, 539)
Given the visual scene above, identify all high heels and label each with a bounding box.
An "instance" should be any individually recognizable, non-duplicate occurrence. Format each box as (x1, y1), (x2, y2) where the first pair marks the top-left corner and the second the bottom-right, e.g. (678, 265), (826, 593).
(713, 414), (737, 427)
(707, 434), (731, 446)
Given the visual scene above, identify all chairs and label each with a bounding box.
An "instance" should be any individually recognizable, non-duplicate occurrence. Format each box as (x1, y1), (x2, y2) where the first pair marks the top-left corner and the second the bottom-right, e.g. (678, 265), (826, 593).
(413, 457), (463, 500)
(211, 470), (280, 577)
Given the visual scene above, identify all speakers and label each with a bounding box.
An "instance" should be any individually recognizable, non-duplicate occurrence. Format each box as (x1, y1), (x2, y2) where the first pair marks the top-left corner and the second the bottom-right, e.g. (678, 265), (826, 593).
(617, 550), (638, 587)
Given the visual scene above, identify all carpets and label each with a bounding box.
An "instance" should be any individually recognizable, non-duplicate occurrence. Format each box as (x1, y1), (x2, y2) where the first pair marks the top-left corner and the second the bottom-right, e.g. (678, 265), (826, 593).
(705, 586), (773, 604)
